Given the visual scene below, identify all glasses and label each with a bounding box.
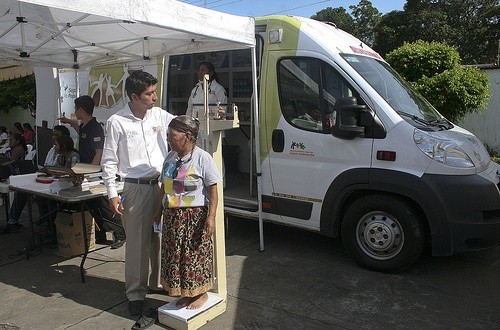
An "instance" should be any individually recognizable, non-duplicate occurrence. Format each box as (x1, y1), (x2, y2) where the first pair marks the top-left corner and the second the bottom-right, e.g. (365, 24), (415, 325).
(172, 158), (181, 179)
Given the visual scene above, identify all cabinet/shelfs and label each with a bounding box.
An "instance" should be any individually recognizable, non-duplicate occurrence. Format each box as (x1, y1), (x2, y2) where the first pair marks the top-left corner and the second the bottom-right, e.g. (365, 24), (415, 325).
(173, 49), (254, 128)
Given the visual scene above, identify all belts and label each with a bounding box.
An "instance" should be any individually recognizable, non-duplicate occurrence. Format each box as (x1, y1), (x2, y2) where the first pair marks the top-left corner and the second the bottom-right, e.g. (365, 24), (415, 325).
(125, 177), (159, 185)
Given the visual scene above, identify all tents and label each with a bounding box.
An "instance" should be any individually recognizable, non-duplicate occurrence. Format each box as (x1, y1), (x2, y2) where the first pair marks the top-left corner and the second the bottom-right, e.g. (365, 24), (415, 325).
(0, 0), (264, 252)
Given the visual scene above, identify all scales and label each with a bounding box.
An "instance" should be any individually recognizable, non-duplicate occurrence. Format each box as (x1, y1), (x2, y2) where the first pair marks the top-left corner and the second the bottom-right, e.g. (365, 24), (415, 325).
(157, 74), (238, 330)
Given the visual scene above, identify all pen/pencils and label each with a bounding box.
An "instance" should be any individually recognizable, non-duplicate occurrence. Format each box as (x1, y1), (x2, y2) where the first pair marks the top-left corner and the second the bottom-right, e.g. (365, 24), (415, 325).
(112, 195), (128, 219)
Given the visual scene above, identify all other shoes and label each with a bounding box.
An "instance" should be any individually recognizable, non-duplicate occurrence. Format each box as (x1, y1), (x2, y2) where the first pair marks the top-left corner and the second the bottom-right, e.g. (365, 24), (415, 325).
(111, 240), (126, 249)
(129, 301), (144, 316)
(0, 223), (20, 233)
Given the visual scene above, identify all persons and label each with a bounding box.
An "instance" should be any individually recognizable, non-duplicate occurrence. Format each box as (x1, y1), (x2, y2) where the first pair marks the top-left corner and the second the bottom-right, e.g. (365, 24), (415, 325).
(46, 135), (80, 175)
(151, 115), (222, 310)
(0, 120), (36, 143)
(2, 124), (70, 232)
(100, 70), (226, 315)
(187, 60), (228, 190)
(5, 133), (28, 160)
(95, 195), (127, 248)
(58, 95), (105, 164)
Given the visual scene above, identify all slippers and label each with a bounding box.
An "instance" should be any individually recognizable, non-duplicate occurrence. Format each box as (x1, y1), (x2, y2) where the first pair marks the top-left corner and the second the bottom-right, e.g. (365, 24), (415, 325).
(131, 314), (158, 330)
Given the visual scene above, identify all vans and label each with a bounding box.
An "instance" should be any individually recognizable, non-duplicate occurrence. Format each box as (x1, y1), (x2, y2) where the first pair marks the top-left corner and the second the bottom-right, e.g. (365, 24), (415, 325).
(36, 15), (500, 272)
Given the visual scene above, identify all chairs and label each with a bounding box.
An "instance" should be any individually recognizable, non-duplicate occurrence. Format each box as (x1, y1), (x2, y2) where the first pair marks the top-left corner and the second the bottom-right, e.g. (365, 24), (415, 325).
(280, 73), (318, 119)
(0, 144), (37, 209)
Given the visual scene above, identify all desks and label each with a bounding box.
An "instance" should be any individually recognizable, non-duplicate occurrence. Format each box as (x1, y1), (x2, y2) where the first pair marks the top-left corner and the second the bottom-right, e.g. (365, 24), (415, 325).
(8, 172), (106, 283)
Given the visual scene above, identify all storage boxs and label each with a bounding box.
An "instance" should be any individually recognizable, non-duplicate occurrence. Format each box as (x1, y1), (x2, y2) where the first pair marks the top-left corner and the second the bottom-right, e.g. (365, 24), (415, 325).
(55, 212), (94, 257)
